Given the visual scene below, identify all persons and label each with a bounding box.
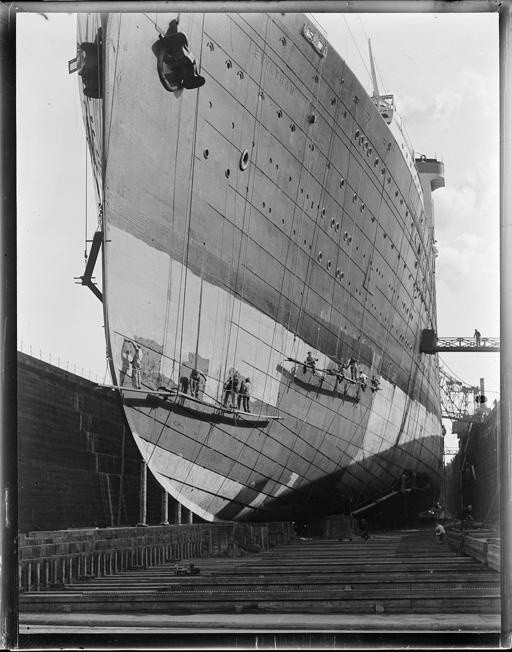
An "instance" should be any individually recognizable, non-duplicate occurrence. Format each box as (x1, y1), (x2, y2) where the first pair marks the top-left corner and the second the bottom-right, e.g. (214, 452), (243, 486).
(301, 351), (318, 376)
(189, 369), (207, 399)
(223, 376), (231, 408)
(236, 379), (244, 409)
(333, 355), (381, 392)
(180, 376), (188, 407)
(119, 339), (129, 386)
(473, 329), (481, 346)
(360, 519), (368, 541)
(434, 520), (448, 545)
(352, 392), (363, 409)
(232, 373), (240, 409)
(241, 377), (252, 414)
(128, 340), (142, 389)
